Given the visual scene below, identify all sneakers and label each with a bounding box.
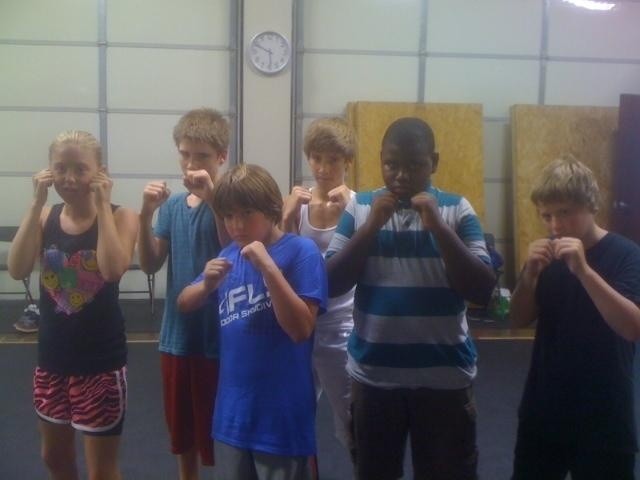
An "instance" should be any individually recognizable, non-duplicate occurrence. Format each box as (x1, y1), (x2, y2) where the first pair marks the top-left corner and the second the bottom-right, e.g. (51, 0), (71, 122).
(14, 303), (40, 333)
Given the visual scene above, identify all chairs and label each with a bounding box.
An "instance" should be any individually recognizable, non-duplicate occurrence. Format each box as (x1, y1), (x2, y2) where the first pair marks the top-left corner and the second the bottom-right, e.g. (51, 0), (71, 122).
(0, 226), (156, 313)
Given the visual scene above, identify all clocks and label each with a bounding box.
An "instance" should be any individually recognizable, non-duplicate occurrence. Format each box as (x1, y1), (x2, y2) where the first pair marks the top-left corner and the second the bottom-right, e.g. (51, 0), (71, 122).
(250, 30), (292, 74)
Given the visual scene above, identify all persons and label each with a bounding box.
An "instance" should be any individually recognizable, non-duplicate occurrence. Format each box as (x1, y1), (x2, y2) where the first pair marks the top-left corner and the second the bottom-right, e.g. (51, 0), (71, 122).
(4, 129), (140, 480)
(508, 154), (640, 479)
(139, 106), (232, 480)
(322, 117), (498, 479)
(174, 162), (329, 480)
(274, 113), (357, 478)
